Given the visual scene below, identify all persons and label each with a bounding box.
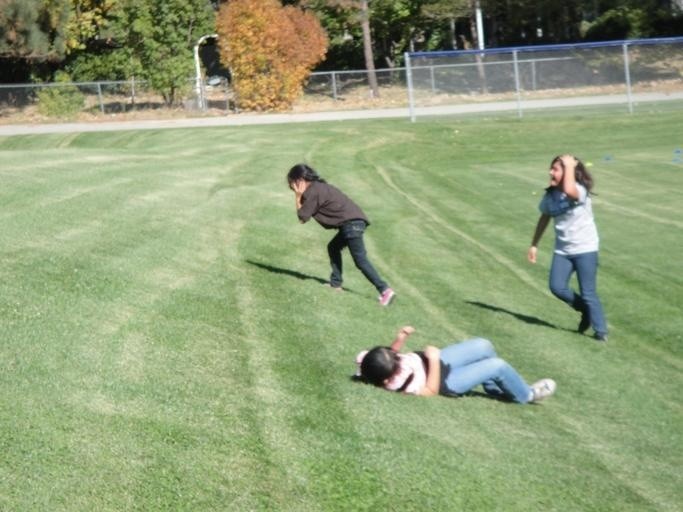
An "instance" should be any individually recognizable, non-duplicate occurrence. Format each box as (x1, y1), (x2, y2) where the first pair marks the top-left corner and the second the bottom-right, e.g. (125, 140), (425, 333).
(525, 152), (609, 343)
(353, 323), (557, 405)
(286, 162), (397, 307)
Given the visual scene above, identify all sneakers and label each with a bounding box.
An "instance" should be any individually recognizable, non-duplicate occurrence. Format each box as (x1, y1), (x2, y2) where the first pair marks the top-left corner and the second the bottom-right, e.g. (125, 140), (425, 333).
(523, 378), (557, 402)
(324, 282), (344, 294)
(578, 316), (611, 342)
(379, 288), (396, 306)
